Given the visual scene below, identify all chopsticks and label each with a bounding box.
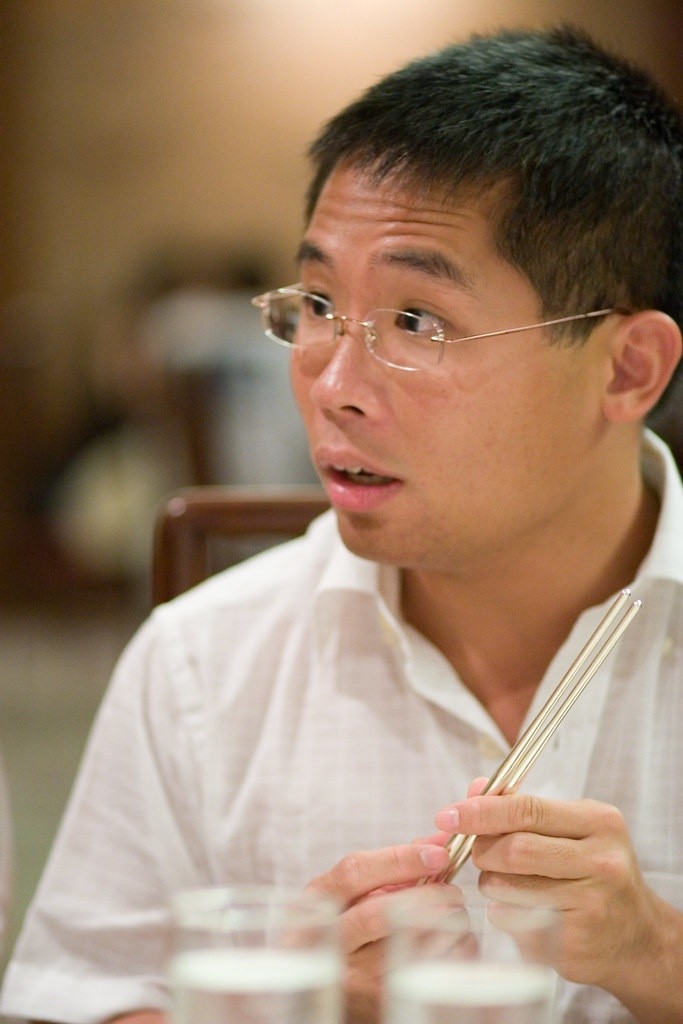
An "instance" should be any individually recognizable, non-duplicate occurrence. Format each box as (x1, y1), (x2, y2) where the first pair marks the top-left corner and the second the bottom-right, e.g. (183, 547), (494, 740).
(418, 590), (643, 889)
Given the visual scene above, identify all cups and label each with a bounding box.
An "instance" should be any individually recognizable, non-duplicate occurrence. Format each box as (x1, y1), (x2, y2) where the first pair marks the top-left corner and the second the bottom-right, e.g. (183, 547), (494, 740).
(378, 887), (566, 1023)
(164, 882), (347, 1023)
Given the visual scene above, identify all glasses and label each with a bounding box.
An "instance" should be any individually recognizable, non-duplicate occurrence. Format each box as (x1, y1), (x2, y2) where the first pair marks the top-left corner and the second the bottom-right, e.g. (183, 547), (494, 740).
(253, 279), (630, 374)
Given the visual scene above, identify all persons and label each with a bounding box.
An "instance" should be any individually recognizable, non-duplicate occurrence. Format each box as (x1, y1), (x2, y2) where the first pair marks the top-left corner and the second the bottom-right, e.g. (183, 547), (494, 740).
(1, 20), (683, 1024)
(53, 265), (322, 579)
